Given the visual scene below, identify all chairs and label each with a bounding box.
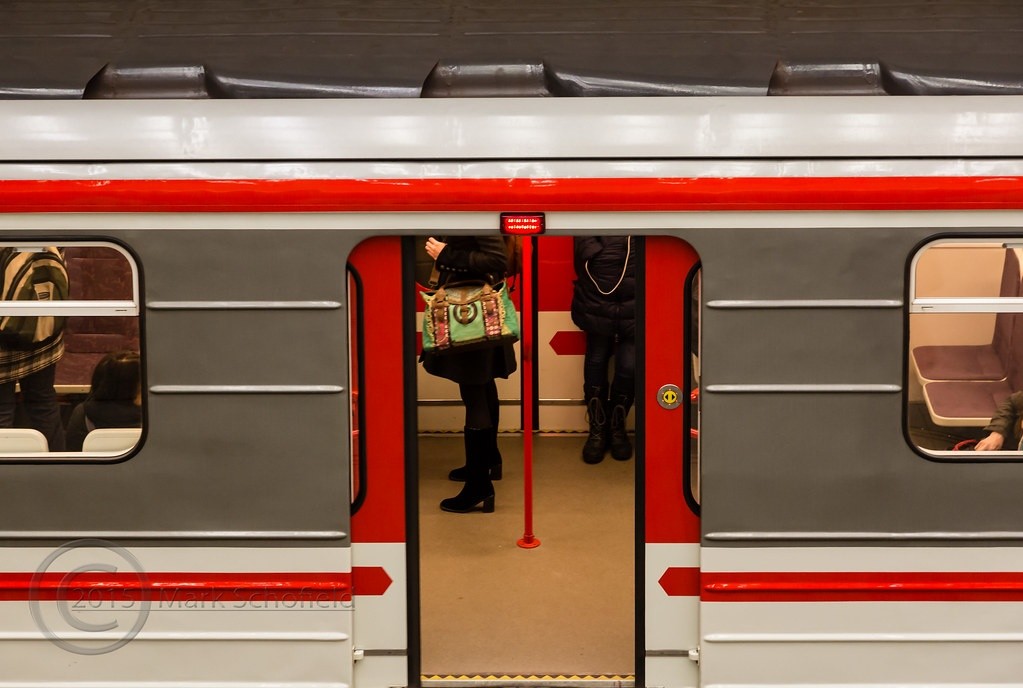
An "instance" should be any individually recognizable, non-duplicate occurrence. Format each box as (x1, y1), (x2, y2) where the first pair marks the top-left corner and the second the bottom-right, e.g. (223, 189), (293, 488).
(50, 244), (139, 397)
(80, 426), (143, 452)
(921, 278), (1023, 428)
(911, 248), (1023, 382)
(0, 427), (51, 455)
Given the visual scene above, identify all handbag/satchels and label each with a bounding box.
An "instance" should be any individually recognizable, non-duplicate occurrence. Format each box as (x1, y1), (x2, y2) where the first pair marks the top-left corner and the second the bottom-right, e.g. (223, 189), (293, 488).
(419, 271), (524, 356)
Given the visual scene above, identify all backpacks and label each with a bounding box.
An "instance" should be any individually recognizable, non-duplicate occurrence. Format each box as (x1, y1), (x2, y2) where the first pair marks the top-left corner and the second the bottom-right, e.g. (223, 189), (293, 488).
(0, 244), (72, 348)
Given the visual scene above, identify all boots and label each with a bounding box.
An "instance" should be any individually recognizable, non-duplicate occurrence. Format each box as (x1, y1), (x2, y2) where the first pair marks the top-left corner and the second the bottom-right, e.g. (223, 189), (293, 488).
(441, 427), (496, 515)
(451, 424), (502, 481)
(583, 394), (610, 464)
(608, 396), (634, 459)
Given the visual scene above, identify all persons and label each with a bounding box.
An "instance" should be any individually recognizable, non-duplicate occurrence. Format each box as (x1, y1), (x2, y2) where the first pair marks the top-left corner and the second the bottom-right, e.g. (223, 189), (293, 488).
(419, 235), (527, 511)
(0, 245), (72, 452)
(571, 233), (636, 464)
(66, 350), (143, 454)
(968, 378), (1023, 453)
